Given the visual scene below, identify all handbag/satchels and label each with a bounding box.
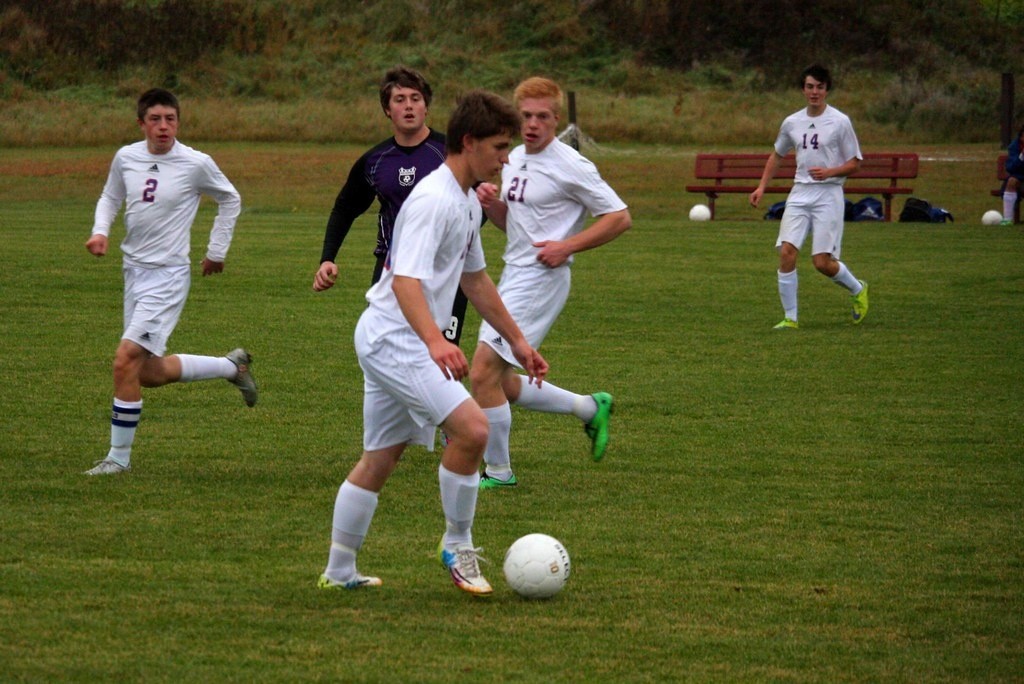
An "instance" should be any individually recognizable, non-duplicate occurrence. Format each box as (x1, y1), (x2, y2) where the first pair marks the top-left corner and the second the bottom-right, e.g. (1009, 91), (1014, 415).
(898, 197), (954, 223)
(765, 197), (883, 221)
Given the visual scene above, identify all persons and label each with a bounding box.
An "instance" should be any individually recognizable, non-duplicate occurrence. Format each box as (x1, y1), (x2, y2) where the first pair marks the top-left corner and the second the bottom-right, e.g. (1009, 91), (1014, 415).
(466, 71), (633, 490)
(317, 89), (550, 594)
(998, 126), (1024, 225)
(79, 92), (257, 477)
(747, 63), (871, 328)
(310, 62), (485, 448)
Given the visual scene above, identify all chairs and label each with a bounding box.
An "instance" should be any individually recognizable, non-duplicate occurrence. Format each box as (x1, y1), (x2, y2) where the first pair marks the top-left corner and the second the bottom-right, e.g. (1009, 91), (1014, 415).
(989, 152), (1024, 225)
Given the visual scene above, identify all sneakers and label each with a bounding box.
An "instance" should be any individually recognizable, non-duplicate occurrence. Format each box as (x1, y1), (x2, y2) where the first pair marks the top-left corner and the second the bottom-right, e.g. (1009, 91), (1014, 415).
(584, 391), (615, 463)
(436, 532), (494, 597)
(1000, 220), (1014, 227)
(478, 470), (517, 491)
(849, 279), (869, 325)
(771, 318), (798, 331)
(226, 347), (258, 409)
(317, 570), (382, 590)
(82, 456), (131, 475)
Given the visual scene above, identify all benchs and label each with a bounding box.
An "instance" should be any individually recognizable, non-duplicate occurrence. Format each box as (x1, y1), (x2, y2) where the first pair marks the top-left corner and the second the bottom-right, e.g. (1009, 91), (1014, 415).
(685, 152), (919, 223)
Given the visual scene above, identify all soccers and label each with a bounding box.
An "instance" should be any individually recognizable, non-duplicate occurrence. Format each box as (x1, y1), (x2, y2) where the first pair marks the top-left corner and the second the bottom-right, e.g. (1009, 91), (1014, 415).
(981, 210), (1003, 225)
(501, 532), (571, 599)
(688, 204), (711, 221)
